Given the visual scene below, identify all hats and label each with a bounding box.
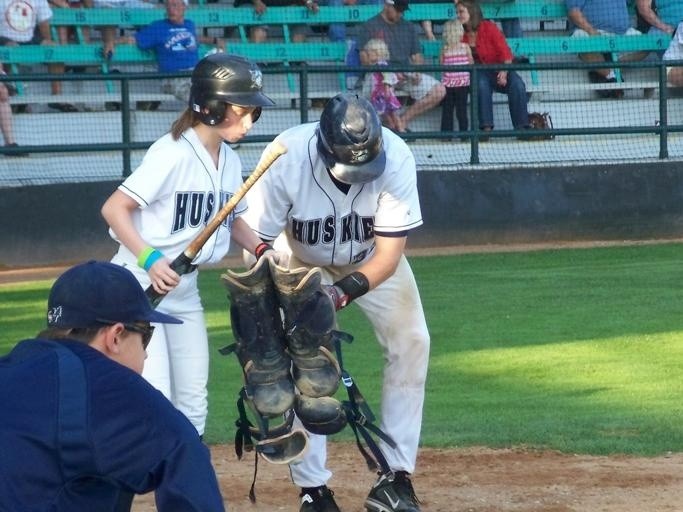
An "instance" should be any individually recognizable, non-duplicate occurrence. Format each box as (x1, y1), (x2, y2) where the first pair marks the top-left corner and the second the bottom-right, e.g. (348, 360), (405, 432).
(48, 259), (184, 330)
(385, 0), (411, 12)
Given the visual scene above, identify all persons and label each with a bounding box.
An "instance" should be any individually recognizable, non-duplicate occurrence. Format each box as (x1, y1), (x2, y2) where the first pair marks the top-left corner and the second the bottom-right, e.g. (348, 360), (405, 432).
(0, 0), (356, 155)
(567, 0), (683, 99)
(99, 55), (287, 436)
(1, 260), (224, 511)
(241, 94), (431, 511)
(346, 1), (534, 155)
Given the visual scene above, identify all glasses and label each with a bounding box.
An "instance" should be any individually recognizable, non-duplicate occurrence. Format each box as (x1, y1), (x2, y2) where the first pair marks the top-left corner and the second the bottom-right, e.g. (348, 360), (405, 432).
(94, 316), (155, 348)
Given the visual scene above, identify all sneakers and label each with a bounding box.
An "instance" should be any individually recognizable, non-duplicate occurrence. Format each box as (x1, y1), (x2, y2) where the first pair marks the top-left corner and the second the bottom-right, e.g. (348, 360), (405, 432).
(608, 75), (624, 98)
(299, 486), (340, 511)
(363, 470), (420, 512)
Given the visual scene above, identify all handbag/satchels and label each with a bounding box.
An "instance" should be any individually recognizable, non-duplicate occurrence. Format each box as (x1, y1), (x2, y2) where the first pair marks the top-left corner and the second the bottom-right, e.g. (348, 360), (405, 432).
(526, 111), (555, 139)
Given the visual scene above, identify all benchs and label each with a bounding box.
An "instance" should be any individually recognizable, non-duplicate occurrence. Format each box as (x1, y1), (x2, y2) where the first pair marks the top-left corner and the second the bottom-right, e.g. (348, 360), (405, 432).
(0, 0), (682, 151)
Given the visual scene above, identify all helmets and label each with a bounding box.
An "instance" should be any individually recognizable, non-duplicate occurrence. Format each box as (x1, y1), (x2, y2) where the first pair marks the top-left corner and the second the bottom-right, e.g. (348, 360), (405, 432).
(187, 53), (276, 126)
(315, 91), (386, 186)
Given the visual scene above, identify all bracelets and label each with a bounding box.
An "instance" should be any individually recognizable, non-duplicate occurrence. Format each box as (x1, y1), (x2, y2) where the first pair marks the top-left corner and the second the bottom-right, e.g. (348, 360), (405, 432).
(144, 250), (162, 271)
(334, 271), (369, 303)
(255, 243), (274, 261)
(137, 247), (154, 267)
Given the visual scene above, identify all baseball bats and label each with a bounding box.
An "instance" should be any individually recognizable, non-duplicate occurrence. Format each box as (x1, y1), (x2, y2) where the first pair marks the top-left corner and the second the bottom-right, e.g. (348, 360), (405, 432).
(143, 140), (289, 308)
